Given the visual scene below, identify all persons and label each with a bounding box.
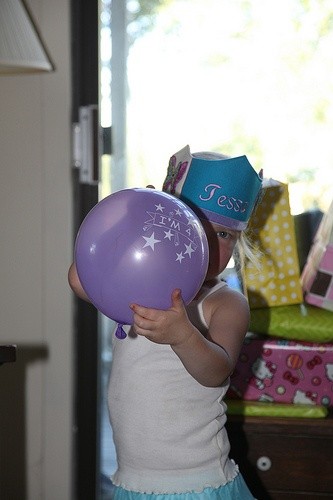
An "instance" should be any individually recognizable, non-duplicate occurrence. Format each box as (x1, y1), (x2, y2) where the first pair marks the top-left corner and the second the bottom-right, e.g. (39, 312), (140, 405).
(69, 144), (256, 500)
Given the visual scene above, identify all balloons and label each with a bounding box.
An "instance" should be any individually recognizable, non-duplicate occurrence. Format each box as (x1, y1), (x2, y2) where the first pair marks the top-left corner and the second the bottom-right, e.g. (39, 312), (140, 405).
(74, 187), (211, 326)
